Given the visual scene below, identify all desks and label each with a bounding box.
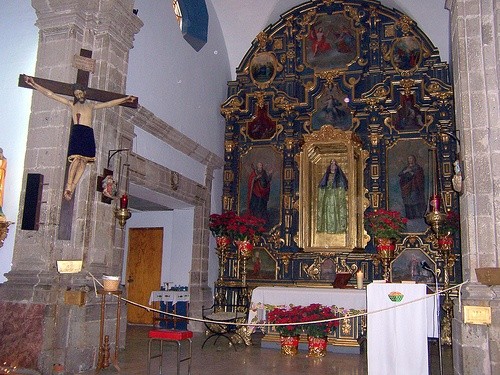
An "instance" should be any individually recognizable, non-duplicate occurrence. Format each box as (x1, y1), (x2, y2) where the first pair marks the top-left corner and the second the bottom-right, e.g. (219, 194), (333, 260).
(247, 285), (440, 348)
(148, 290), (189, 330)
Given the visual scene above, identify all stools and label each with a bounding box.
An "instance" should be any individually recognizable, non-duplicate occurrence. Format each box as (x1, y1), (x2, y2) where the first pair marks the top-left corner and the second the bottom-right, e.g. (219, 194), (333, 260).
(146, 328), (193, 375)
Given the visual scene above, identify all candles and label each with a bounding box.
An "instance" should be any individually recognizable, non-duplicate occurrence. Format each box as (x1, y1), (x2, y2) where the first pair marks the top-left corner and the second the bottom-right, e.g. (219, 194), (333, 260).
(356, 270), (364, 288)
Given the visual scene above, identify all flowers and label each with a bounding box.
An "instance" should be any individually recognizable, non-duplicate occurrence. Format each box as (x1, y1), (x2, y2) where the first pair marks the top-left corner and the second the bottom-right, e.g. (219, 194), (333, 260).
(267, 302), (339, 338)
(209, 211), (266, 239)
(364, 208), (407, 240)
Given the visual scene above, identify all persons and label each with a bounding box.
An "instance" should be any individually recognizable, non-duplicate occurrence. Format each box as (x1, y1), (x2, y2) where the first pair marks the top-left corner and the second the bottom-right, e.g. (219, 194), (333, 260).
(22, 74), (137, 200)
(317, 159), (348, 234)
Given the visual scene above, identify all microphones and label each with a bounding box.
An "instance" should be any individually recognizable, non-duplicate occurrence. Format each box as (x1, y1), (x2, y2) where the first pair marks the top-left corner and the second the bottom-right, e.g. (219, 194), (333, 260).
(421, 260), (431, 269)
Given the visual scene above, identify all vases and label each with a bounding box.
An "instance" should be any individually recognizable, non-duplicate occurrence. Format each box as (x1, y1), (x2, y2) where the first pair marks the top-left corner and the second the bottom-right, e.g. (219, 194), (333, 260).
(240, 242), (253, 259)
(307, 335), (328, 357)
(217, 236), (233, 247)
(280, 335), (300, 356)
(376, 237), (394, 259)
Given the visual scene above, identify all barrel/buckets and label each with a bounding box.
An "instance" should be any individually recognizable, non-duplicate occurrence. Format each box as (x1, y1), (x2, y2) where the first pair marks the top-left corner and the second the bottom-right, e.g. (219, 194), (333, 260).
(103, 276), (119, 291)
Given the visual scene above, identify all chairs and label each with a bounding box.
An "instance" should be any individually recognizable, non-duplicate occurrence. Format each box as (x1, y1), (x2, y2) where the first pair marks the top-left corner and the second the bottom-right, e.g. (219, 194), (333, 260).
(200, 302), (249, 351)
